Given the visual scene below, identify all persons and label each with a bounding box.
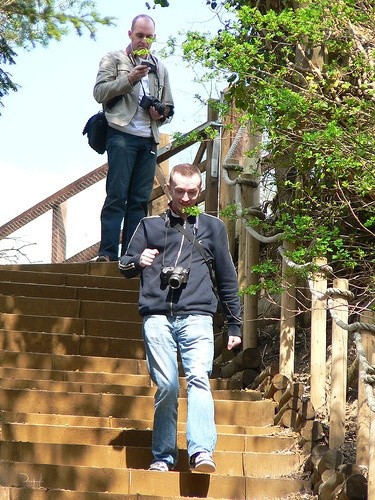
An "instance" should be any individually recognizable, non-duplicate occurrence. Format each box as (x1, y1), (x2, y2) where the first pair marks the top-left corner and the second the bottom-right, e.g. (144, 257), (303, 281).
(92, 14), (175, 263)
(117, 163), (243, 474)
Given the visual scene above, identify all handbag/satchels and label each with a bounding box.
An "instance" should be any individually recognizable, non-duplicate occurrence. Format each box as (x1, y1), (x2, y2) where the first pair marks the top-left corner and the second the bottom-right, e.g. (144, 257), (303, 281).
(83, 111), (108, 154)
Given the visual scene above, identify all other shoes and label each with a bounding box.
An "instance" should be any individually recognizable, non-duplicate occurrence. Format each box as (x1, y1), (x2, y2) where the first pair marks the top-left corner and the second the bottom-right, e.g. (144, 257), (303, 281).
(96, 254), (109, 262)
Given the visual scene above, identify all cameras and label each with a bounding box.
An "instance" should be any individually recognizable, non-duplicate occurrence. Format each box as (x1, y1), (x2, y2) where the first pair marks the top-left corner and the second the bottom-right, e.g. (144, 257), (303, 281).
(161, 266), (190, 289)
(139, 95), (174, 119)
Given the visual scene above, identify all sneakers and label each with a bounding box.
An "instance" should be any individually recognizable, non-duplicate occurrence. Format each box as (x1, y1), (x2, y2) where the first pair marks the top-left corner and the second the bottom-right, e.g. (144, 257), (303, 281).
(189, 452), (216, 472)
(149, 462), (169, 471)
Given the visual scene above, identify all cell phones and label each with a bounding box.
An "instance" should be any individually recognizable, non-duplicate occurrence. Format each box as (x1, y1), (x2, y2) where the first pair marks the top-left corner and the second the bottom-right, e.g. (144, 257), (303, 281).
(141, 61), (149, 67)
